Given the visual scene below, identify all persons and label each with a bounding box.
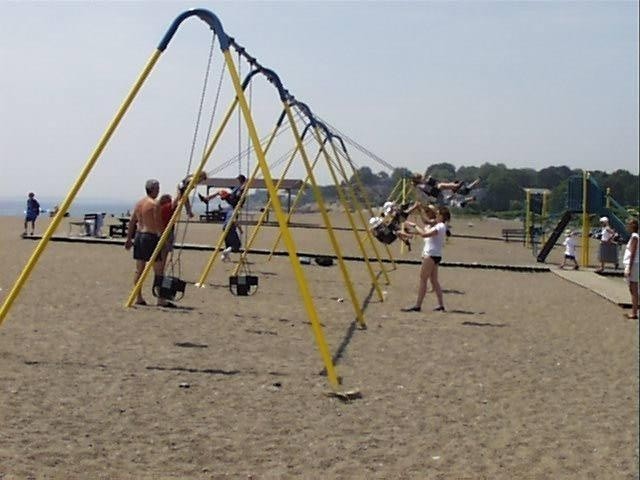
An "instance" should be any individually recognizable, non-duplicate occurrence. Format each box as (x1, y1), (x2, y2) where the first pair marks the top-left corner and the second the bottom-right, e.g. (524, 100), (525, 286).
(623, 218), (639, 319)
(399, 207), (452, 312)
(177, 170), (209, 219)
(196, 172), (246, 208)
(219, 205), (245, 262)
(18, 190), (42, 236)
(123, 177), (176, 308)
(368, 172), (486, 252)
(151, 189), (182, 296)
(592, 216), (621, 275)
(560, 230), (581, 271)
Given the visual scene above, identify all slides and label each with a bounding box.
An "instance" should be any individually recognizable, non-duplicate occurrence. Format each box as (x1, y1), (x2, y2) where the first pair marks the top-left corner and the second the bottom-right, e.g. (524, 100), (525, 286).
(605, 210), (631, 243)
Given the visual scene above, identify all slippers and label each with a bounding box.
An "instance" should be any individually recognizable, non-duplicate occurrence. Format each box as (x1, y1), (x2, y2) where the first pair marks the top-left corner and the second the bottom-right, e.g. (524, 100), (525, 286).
(401, 305), (445, 312)
(137, 299), (178, 308)
(624, 313), (639, 321)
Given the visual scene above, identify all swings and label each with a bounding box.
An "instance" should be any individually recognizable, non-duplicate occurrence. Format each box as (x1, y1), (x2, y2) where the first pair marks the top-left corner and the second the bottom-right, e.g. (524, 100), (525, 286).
(153, 32), (451, 307)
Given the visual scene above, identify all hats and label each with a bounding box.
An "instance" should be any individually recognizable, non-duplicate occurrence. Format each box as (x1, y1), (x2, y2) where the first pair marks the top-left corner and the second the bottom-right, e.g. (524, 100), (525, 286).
(369, 218), (383, 230)
(600, 216), (609, 222)
(564, 230), (573, 236)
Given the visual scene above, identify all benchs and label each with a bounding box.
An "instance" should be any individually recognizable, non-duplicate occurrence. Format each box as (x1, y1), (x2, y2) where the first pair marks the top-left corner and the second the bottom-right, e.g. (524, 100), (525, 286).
(502, 229), (540, 242)
